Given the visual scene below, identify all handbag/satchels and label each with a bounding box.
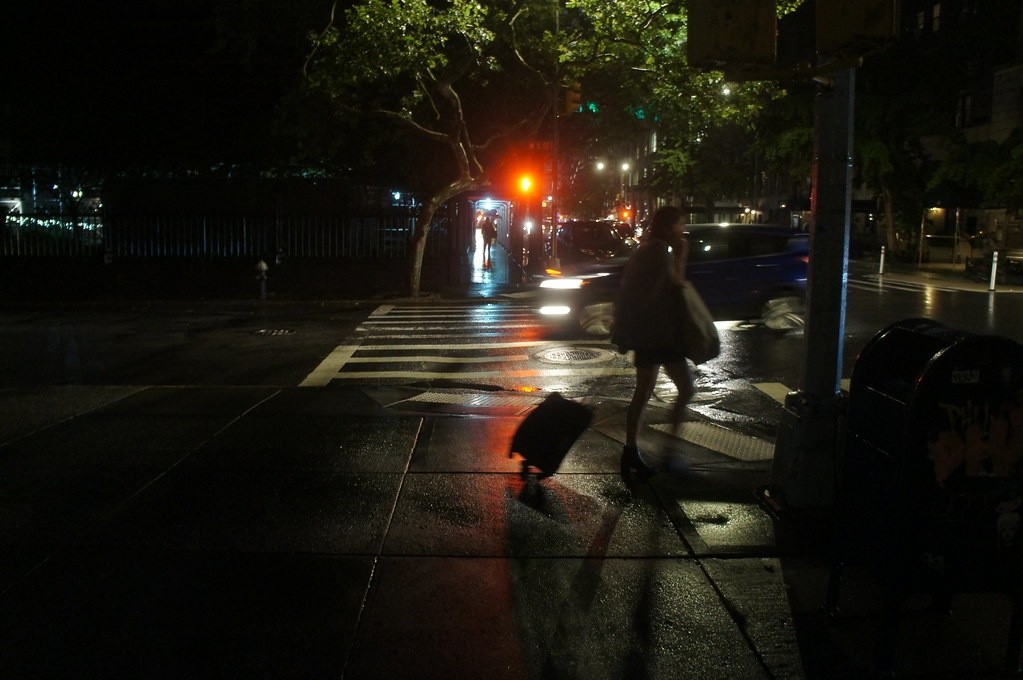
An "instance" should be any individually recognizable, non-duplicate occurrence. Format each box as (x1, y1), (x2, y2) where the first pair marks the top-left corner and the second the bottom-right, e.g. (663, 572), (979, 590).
(676, 276), (721, 364)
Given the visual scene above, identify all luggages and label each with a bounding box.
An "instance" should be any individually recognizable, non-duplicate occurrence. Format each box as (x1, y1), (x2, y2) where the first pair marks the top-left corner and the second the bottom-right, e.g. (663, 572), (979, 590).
(509, 352), (622, 479)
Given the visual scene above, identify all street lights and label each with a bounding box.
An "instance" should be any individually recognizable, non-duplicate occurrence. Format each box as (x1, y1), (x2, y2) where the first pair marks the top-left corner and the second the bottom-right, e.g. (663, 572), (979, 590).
(593, 161), (631, 221)
(745, 208), (750, 224)
(518, 173), (536, 278)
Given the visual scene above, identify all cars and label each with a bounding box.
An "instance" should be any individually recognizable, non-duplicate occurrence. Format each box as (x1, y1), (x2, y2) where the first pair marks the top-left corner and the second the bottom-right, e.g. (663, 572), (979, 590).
(575, 221), (811, 339)
(545, 220), (634, 271)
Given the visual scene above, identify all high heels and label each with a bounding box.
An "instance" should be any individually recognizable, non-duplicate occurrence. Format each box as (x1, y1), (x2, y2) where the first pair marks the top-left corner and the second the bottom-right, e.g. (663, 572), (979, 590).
(620, 446), (660, 476)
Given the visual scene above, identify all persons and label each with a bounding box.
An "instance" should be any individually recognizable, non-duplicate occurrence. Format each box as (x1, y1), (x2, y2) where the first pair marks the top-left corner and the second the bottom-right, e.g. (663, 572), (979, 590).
(482, 216), (495, 258)
(615, 207), (697, 476)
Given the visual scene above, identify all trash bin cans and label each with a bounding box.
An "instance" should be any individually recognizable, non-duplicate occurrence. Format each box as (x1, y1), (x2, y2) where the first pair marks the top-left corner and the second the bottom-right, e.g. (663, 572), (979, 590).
(824, 318), (1023, 678)
